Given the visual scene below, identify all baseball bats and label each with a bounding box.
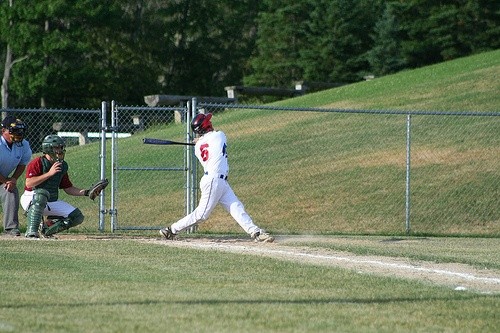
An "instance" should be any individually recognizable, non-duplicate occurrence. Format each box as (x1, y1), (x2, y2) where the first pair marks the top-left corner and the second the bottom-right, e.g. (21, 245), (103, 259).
(143, 138), (195, 146)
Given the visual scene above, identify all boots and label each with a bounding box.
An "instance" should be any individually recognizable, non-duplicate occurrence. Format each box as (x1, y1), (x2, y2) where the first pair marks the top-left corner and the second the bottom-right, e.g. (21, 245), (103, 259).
(45, 218), (74, 236)
(25, 205), (44, 238)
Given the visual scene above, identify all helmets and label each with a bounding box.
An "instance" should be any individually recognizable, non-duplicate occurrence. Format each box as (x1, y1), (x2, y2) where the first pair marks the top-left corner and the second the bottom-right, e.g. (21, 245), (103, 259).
(42, 135), (66, 163)
(191, 113), (213, 137)
(0, 116), (26, 147)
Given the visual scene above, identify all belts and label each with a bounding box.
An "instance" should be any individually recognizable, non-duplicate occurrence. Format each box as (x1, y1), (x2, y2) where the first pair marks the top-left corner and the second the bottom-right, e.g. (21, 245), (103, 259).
(205, 171), (228, 180)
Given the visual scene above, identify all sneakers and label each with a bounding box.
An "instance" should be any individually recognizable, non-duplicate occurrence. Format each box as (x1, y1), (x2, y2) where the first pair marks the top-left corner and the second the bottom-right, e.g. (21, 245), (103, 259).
(251, 229), (275, 243)
(159, 227), (178, 240)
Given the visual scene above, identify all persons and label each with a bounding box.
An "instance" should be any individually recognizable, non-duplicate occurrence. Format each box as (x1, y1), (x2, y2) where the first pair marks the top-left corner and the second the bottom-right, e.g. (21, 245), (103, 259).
(20, 134), (109, 240)
(159, 113), (275, 244)
(0, 116), (32, 235)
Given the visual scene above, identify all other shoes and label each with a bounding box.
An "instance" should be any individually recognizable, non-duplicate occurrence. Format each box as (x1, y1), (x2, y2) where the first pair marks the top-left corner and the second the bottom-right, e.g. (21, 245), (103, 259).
(7, 228), (20, 236)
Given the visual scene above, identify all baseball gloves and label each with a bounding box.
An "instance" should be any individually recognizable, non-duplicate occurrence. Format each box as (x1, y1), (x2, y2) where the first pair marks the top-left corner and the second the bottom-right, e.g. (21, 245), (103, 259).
(85, 178), (109, 200)
